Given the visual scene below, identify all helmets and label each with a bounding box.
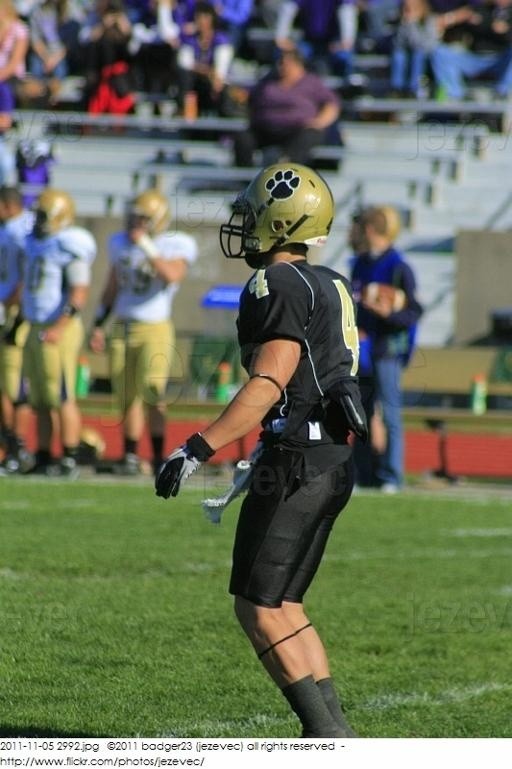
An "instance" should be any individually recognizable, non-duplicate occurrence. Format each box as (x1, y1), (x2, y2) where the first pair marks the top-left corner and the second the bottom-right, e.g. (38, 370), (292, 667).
(123, 191), (172, 241)
(29, 189), (80, 237)
(351, 205), (401, 245)
(214, 161), (337, 266)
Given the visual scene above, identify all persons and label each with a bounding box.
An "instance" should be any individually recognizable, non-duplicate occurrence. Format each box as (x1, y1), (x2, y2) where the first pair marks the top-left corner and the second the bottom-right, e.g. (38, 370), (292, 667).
(87, 190), (201, 478)
(342, 205), (423, 492)
(154, 157), (373, 738)
(6, 189), (99, 479)
(0, 186), (37, 478)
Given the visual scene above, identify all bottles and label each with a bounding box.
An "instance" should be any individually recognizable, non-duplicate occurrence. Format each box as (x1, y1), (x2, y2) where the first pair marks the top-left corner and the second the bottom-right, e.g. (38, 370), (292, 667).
(468, 373), (488, 416)
(76, 355), (91, 400)
(214, 362), (233, 406)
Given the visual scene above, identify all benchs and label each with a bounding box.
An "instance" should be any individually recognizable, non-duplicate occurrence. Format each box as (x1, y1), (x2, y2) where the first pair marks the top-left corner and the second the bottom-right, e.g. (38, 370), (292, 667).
(0, 0), (512, 479)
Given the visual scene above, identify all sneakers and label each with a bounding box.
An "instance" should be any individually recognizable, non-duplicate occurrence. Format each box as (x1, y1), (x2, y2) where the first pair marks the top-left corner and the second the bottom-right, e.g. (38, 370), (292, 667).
(0, 444), (142, 481)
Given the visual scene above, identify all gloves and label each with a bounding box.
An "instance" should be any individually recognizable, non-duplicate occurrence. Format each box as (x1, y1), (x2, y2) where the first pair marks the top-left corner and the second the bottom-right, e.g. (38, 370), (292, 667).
(150, 430), (218, 500)
